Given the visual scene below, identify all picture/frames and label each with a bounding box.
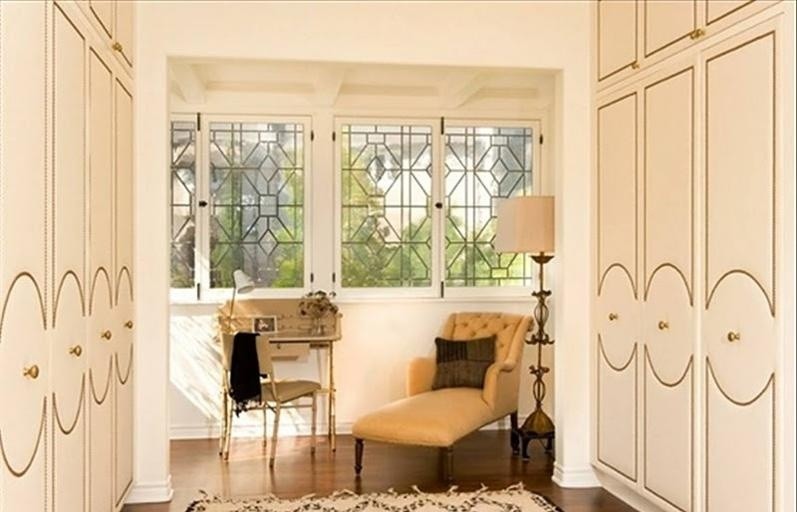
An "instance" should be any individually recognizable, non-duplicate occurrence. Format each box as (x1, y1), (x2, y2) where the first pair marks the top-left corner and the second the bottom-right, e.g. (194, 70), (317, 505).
(251, 315), (278, 336)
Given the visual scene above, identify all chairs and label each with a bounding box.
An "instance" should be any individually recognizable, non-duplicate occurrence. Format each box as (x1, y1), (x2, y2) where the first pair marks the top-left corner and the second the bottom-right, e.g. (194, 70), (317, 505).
(222, 330), (321, 463)
(351, 310), (536, 485)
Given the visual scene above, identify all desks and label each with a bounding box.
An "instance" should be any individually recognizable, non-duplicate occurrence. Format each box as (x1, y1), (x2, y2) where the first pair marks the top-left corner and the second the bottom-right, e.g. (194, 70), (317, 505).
(217, 298), (343, 451)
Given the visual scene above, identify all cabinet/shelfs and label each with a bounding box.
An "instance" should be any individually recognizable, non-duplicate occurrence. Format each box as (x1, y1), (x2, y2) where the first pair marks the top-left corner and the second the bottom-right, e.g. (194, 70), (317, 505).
(588, 0), (796, 512)
(4, 1), (135, 512)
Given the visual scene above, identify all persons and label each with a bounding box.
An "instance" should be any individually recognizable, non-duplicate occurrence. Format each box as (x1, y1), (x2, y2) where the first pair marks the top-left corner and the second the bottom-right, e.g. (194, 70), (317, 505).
(256, 318), (272, 331)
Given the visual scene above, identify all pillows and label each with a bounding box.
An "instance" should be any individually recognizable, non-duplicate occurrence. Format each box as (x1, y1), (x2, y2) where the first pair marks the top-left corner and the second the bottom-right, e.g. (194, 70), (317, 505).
(430, 332), (498, 391)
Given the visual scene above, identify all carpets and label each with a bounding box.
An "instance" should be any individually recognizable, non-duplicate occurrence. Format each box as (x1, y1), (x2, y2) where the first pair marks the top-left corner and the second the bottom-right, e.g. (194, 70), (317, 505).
(183, 482), (565, 512)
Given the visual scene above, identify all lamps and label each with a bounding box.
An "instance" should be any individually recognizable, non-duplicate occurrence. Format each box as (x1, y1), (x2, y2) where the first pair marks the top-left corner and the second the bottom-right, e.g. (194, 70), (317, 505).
(493, 196), (554, 460)
(226, 269), (255, 333)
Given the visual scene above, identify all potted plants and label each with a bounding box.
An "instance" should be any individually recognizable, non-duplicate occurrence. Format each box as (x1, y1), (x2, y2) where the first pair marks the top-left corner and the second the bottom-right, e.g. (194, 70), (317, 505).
(297, 290), (339, 336)
